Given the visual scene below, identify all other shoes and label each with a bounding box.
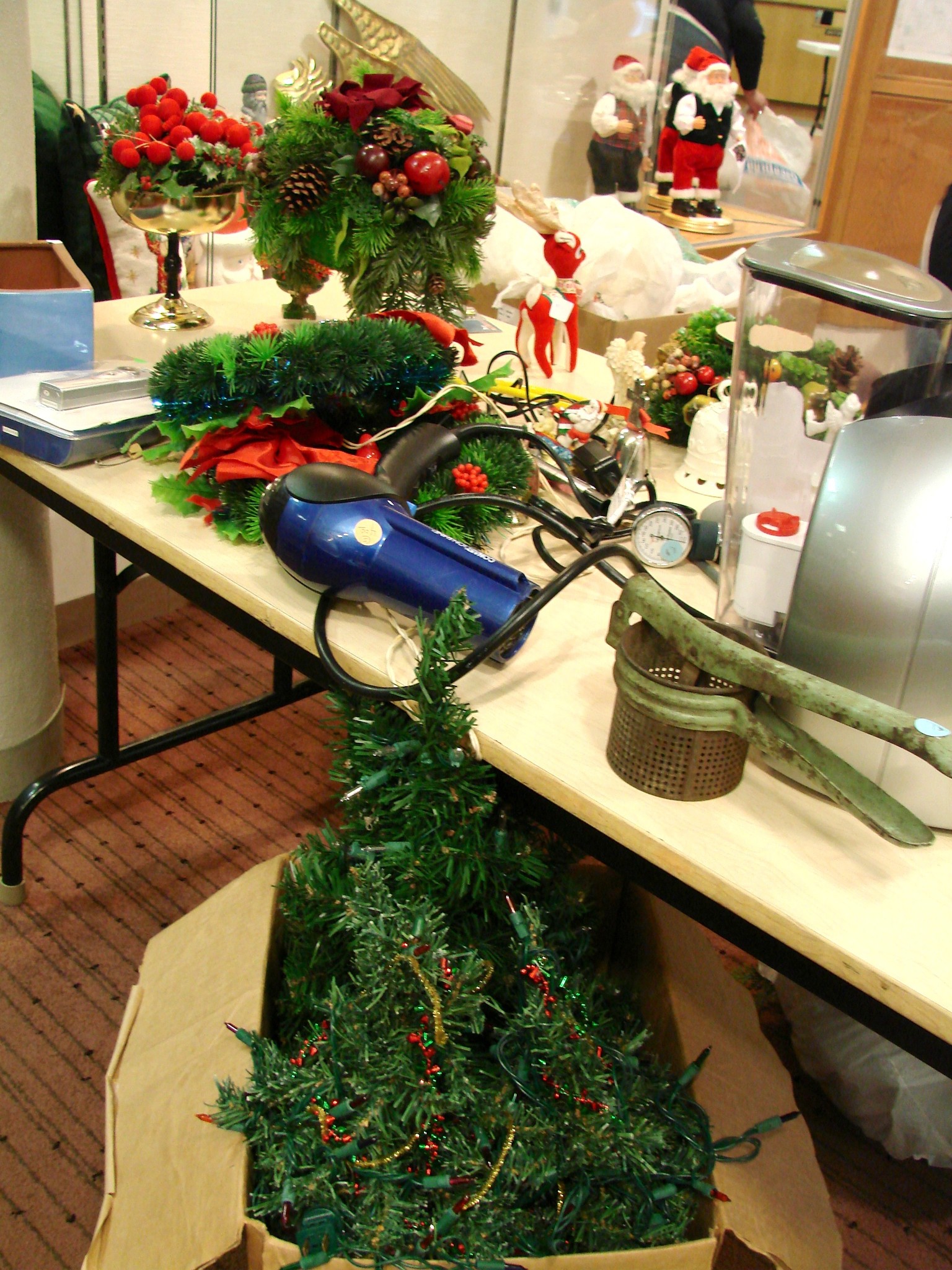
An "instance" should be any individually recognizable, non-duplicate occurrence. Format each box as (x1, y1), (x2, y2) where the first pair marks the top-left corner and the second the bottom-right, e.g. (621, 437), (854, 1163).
(671, 198), (697, 217)
(696, 200), (723, 218)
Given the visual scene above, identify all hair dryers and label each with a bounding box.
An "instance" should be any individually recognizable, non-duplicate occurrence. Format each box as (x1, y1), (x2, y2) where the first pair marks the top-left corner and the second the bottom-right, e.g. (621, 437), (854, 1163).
(253, 422), (544, 669)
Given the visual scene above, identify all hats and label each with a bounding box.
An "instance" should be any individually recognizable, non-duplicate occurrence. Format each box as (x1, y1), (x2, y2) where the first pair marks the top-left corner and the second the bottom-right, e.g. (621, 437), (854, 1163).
(612, 52), (647, 82)
(695, 51), (738, 92)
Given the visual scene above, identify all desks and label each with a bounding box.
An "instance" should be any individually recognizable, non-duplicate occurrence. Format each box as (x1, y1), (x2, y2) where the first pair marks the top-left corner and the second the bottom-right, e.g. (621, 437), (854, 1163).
(797, 38), (842, 138)
(0, 265), (952, 1075)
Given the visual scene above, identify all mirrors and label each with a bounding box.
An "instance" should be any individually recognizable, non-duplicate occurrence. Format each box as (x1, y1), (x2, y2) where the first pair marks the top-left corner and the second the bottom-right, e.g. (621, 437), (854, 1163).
(643, 0), (860, 227)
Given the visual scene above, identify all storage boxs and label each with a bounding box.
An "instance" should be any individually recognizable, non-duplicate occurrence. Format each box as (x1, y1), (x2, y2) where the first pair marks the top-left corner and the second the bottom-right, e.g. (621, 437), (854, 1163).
(0, 241), (94, 380)
(457, 252), (824, 374)
(78, 852), (846, 1270)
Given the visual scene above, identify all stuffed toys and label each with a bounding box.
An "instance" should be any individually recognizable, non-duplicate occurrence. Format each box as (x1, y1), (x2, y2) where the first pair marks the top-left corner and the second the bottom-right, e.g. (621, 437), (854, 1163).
(494, 178), (585, 378)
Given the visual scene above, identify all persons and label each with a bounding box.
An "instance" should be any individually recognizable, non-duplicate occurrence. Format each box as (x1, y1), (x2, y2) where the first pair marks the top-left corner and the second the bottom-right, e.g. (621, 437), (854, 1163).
(655, 47), (714, 195)
(588, 54), (655, 214)
(665, 1), (769, 121)
(670, 53), (747, 217)
